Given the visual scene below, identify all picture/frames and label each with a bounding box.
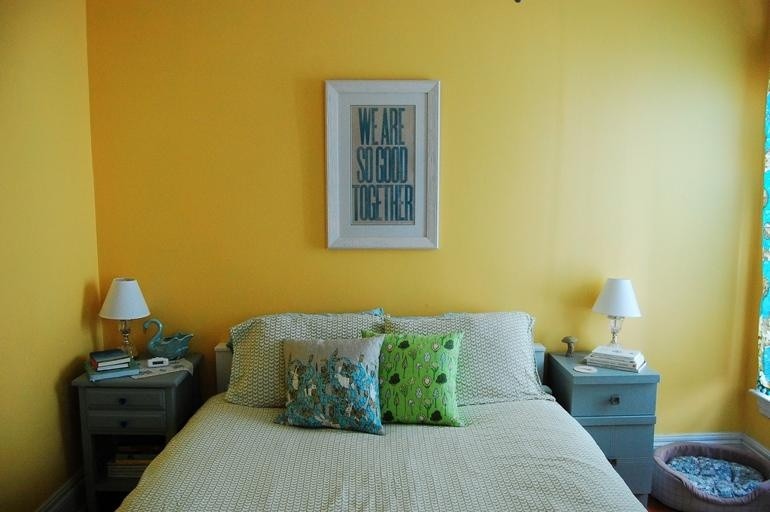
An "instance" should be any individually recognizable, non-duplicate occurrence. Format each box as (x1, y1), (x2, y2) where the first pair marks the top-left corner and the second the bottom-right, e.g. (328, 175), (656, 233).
(325, 79), (439, 250)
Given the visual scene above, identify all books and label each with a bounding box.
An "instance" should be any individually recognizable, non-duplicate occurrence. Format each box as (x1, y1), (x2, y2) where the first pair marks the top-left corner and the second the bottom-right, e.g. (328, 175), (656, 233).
(583, 345), (647, 373)
(102, 441), (164, 482)
(83, 348), (140, 381)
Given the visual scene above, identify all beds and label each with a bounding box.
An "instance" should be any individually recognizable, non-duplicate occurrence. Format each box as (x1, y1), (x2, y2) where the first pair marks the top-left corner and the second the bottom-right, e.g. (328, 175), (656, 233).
(116, 343), (648, 512)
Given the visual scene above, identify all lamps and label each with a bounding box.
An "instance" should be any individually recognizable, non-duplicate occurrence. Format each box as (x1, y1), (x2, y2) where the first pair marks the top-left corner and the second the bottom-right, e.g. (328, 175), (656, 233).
(582, 277), (648, 374)
(97, 277), (150, 360)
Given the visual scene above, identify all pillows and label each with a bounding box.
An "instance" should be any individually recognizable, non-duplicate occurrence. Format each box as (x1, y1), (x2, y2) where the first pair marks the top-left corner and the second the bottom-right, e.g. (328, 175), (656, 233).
(271, 337), (386, 435)
(362, 330), (464, 428)
(226, 312), (390, 408)
(384, 312), (555, 408)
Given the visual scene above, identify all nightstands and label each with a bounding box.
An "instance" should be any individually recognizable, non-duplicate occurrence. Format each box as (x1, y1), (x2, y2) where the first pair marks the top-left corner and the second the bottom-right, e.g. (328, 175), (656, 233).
(71, 352), (205, 512)
(546, 352), (660, 510)
